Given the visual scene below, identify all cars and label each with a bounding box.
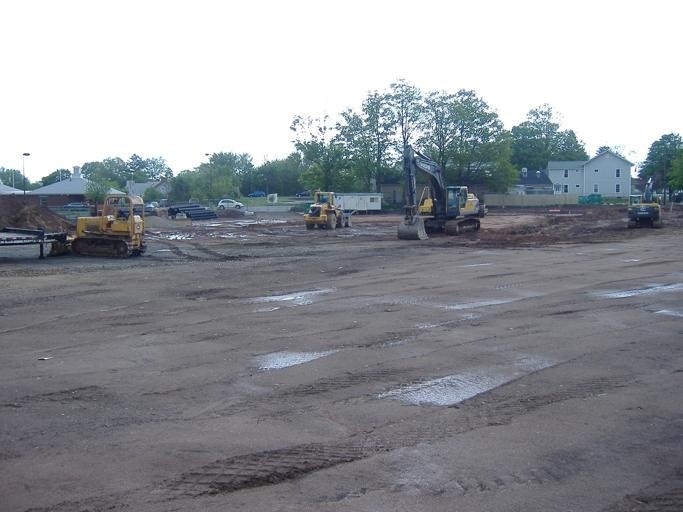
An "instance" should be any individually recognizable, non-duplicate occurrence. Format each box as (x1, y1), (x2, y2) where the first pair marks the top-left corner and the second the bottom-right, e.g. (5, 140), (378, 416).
(63, 202), (87, 211)
(217, 199), (243, 208)
(248, 191), (265, 197)
(296, 191), (310, 198)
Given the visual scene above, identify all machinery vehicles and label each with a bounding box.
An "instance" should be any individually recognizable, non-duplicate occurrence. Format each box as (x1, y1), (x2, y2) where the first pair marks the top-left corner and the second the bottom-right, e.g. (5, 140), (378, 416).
(304, 192), (352, 228)
(397, 144), (488, 240)
(626, 177), (663, 228)
(43, 194), (146, 258)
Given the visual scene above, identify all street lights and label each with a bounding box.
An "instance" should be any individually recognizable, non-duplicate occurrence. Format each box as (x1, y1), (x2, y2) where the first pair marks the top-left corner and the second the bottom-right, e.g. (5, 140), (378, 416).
(206, 154), (212, 193)
(23, 152), (30, 196)
